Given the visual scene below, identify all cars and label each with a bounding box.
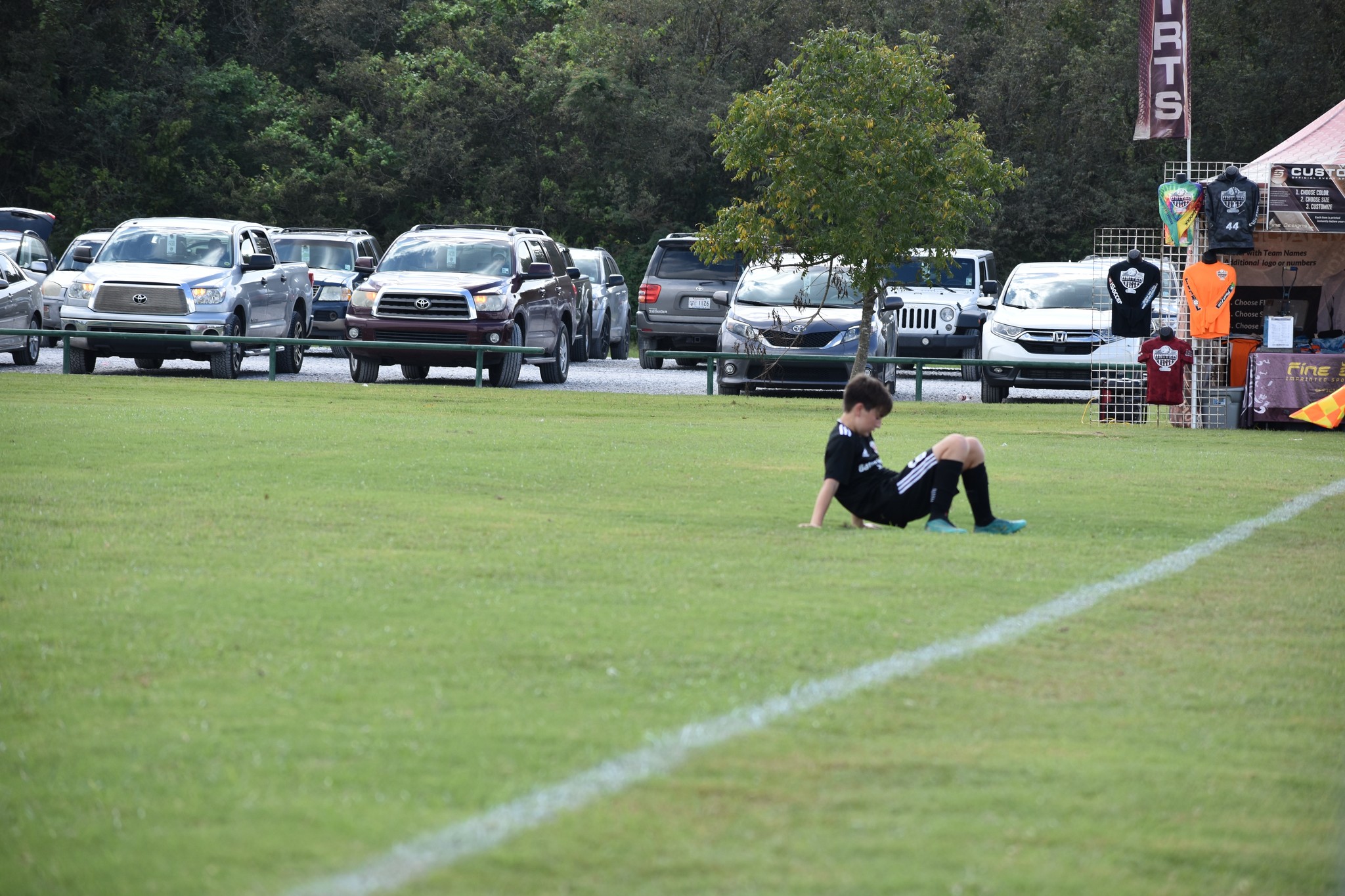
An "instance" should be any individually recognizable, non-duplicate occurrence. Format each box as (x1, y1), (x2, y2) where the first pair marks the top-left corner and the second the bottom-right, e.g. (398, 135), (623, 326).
(712, 253), (904, 395)
(975, 261), (1155, 404)
(0, 249), (44, 366)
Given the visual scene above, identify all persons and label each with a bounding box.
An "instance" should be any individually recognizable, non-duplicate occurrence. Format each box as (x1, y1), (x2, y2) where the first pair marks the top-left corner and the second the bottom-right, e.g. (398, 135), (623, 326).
(797, 372), (1028, 536)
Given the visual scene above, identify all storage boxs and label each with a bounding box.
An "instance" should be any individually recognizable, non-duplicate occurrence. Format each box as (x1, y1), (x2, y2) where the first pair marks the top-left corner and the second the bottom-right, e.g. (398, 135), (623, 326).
(1261, 314), (1295, 351)
(1198, 383), (1245, 431)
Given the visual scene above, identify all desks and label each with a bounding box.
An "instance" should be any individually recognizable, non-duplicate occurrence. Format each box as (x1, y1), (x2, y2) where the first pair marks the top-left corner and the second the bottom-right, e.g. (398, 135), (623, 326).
(1243, 346), (1345, 434)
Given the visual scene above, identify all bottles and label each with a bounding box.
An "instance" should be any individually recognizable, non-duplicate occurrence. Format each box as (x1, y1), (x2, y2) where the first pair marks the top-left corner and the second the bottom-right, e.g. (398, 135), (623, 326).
(957, 394), (972, 401)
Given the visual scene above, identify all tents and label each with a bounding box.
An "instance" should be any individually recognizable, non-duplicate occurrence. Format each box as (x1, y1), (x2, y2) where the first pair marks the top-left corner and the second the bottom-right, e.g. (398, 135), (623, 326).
(1191, 99), (1345, 429)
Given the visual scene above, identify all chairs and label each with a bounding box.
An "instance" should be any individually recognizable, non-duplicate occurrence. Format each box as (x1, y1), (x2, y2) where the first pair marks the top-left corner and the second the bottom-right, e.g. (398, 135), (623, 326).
(665, 254), (744, 281)
(755, 270), (859, 303)
(889, 260), (971, 289)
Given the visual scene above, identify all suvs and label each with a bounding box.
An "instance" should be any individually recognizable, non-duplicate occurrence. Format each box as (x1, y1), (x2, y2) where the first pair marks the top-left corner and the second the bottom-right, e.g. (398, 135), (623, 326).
(344, 224), (575, 389)
(0, 206), (58, 288)
(635, 233), (784, 369)
(40, 228), (115, 348)
(881, 249), (999, 381)
(269, 227), (384, 359)
(568, 246), (632, 359)
(1075, 255), (1181, 338)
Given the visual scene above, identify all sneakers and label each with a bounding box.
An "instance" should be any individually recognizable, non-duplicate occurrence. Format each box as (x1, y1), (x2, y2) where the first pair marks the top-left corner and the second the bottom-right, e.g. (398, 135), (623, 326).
(923, 518), (967, 534)
(973, 518), (1027, 535)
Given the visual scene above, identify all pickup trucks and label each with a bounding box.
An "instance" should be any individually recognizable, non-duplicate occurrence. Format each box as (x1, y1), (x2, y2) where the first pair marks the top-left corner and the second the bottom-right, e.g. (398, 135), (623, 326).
(556, 242), (593, 362)
(59, 216), (314, 380)
